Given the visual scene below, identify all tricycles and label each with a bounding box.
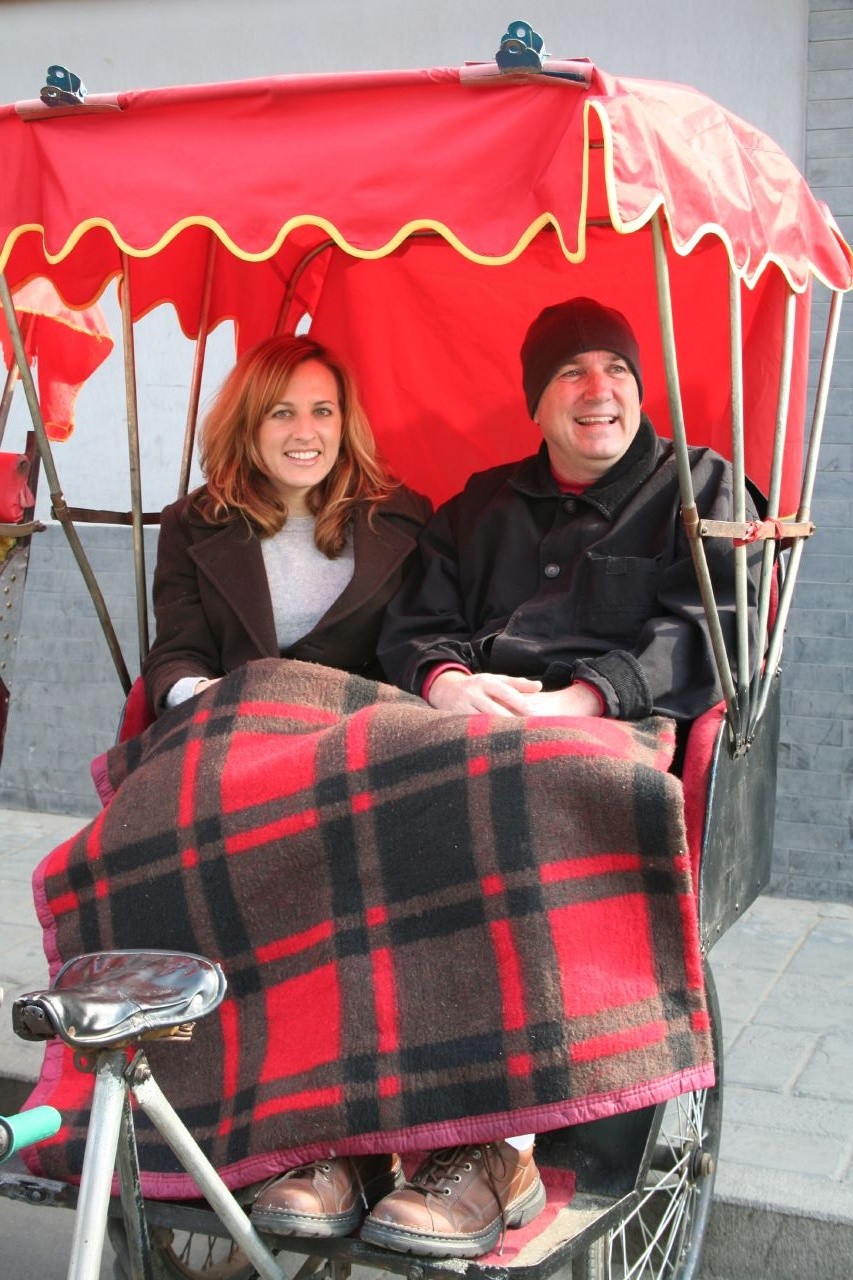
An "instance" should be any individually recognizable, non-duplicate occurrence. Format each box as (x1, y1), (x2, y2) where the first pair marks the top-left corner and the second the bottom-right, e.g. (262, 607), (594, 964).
(2, 17), (853, 1279)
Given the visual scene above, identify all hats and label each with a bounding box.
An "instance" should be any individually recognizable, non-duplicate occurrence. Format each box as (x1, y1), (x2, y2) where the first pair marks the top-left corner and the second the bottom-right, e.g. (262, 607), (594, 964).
(519, 297), (644, 420)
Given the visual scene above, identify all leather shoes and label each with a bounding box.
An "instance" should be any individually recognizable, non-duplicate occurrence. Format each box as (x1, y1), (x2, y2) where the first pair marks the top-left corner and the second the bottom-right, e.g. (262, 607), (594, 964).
(252, 1152), (407, 1239)
(360, 1140), (546, 1258)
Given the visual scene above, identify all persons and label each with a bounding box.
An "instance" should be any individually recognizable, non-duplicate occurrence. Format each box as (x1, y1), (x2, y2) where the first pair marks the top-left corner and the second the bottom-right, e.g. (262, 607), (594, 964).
(248, 298), (770, 1259)
(144, 335), (435, 714)
